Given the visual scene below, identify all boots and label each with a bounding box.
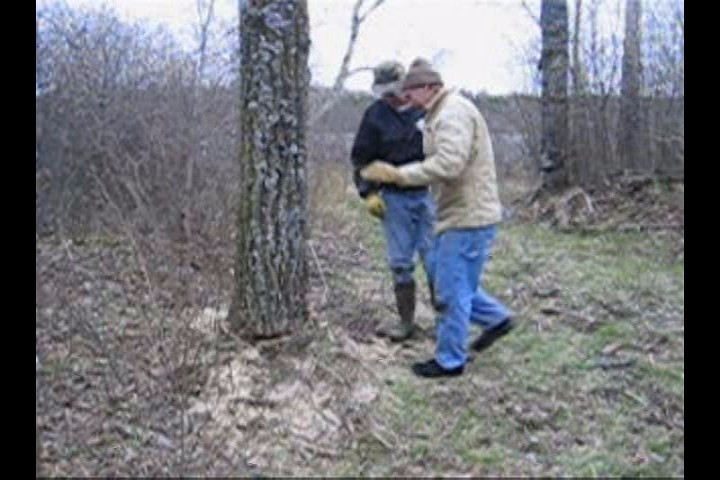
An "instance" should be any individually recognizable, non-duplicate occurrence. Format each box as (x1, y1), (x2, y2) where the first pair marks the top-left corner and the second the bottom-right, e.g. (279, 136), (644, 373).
(389, 284), (415, 341)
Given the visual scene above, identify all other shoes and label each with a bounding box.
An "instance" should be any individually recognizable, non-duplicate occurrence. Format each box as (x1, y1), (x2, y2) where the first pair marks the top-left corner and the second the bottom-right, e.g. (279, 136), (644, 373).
(470, 316), (515, 353)
(412, 359), (463, 378)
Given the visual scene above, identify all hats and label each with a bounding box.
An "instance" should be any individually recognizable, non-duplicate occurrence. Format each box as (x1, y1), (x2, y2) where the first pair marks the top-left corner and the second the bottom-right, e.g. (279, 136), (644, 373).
(369, 61), (404, 93)
(402, 57), (444, 90)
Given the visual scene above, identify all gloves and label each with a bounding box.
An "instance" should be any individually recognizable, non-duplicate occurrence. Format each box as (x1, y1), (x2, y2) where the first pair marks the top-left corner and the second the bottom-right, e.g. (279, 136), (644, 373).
(364, 193), (386, 220)
(360, 160), (398, 183)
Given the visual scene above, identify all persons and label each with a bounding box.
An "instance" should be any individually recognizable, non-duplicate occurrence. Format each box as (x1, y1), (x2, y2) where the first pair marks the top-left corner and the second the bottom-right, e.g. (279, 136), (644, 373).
(350, 57), (518, 379)
